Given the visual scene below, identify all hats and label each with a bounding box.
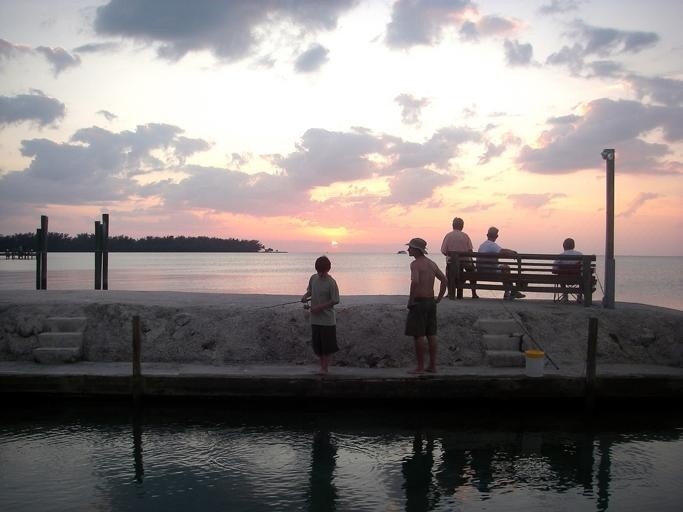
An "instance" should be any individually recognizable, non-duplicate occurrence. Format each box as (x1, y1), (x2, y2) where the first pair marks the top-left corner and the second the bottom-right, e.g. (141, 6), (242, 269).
(404, 238), (429, 255)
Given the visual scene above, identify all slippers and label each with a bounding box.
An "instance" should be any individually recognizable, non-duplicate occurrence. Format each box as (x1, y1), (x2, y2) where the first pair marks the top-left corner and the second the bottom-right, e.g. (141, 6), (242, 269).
(408, 368), (424, 373)
(425, 367), (436, 373)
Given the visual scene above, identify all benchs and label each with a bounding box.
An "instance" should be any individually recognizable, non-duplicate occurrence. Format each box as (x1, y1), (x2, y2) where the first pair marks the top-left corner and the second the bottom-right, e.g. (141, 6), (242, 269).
(445, 250), (598, 307)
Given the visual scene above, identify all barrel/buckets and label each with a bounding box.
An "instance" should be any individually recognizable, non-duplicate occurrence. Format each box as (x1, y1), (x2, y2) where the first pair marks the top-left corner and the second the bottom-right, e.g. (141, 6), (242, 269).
(525, 349), (546, 376)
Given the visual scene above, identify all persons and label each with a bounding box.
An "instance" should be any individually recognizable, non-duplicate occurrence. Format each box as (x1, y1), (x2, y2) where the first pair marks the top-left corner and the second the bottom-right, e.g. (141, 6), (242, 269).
(302, 255), (340, 375)
(405, 238), (448, 374)
(477, 226), (526, 299)
(441, 217), (479, 299)
(551, 238), (584, 303)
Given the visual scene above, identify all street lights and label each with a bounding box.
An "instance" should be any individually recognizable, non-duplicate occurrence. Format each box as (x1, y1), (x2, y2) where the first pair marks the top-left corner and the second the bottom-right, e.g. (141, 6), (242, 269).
(601, 149), (615, 308)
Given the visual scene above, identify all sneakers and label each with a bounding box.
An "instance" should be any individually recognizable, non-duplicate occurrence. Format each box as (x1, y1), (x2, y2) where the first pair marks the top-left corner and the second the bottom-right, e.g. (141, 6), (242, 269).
(511, 291), (526, 298)
(504, 292), (511, 298)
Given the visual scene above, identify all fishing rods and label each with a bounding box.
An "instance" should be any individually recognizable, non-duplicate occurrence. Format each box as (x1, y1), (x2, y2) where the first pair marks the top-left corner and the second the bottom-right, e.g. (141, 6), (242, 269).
(500, 301), (559, 369)
(260, 298), (311, 312)
